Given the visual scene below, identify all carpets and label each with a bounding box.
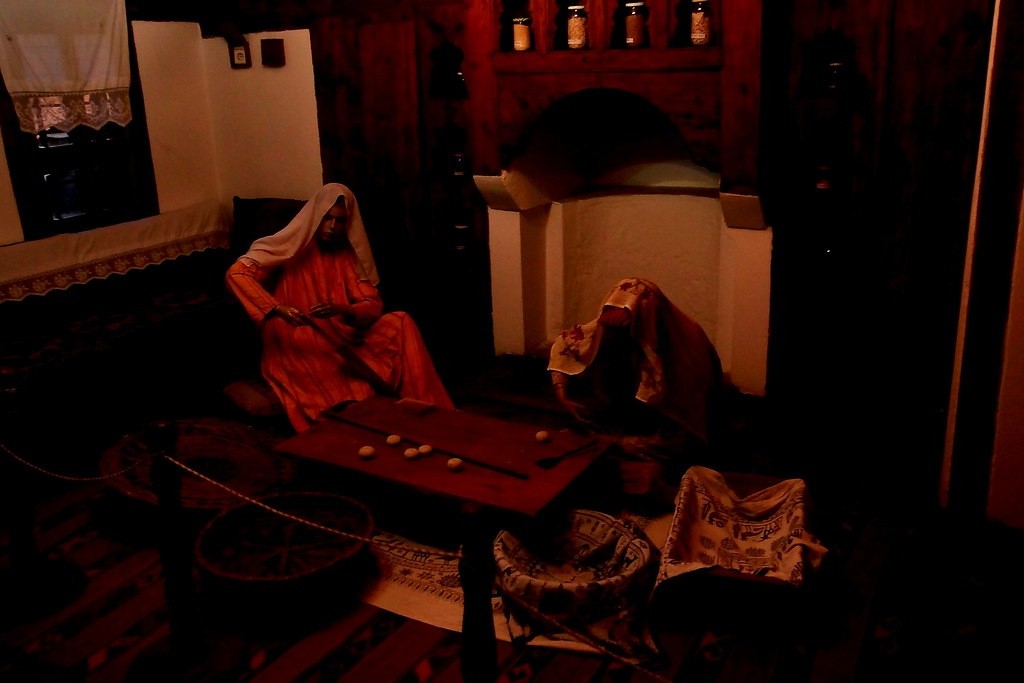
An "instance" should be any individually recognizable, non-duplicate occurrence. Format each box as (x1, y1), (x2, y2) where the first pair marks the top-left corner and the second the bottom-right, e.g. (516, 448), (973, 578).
(347, 476), (677, 655)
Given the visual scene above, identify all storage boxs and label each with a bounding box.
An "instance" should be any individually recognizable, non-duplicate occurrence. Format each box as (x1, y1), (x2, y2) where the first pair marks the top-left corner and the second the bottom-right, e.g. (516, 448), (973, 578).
(96, 416), (294, 538)
(196, 490), (375, 619)
(493, 508), (651, 626)
(649, 464), (831, 623)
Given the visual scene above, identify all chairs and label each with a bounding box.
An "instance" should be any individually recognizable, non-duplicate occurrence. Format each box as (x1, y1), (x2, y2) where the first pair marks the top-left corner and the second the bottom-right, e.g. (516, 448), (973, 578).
(233, 196), (312, 259)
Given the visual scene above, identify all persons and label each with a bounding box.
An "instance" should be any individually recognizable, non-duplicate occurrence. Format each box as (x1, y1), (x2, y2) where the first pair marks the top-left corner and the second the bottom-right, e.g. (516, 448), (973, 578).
(225, 180), (459, 430)
(548, 276), (724, 459)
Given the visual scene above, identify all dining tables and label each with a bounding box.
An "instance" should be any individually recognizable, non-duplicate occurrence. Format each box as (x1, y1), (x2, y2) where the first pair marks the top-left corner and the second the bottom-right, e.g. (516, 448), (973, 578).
(272, 395), (624, 683)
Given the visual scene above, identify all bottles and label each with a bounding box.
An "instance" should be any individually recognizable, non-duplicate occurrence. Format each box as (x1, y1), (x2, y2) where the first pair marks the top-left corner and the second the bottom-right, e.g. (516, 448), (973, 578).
(690, 0), (712, 46)
(512, 17), (533, 51)
(624, 1), (646, 48)
(566, 4), (588, 49)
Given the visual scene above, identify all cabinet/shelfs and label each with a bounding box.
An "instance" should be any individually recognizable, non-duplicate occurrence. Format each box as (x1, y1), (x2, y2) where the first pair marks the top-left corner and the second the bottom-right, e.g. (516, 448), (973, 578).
(463, 0), (762, 191)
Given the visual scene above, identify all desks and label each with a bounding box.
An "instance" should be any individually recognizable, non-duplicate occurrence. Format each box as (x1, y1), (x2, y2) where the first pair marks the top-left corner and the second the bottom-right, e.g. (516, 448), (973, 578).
(1, 201), (229, 341)
